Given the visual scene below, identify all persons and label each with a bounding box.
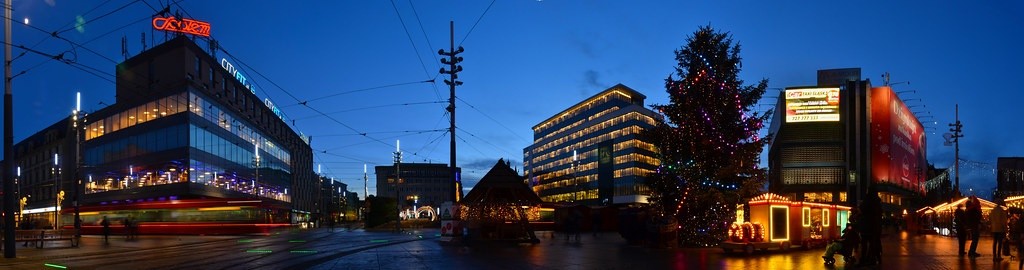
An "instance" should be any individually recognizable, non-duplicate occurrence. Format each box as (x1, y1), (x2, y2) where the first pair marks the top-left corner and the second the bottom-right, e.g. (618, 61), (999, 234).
(955, 195), (1024, 270)
(0, 216), (138, 247)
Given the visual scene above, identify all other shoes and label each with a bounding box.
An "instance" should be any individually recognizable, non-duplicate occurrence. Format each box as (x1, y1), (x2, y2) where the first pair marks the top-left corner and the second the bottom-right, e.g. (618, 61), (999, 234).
(968, 252), (980, 256)
(993, 258), (1004, 262)
(959, 252), (967, 254)
(22, 244), (27, 247)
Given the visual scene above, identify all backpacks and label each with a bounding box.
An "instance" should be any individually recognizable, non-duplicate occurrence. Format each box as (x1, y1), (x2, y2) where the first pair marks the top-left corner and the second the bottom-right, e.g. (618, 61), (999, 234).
(1002, 238), (1010, 256)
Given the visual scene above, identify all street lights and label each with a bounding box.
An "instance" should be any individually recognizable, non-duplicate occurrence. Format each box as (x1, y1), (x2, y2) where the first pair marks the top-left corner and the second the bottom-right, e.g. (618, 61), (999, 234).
(51, 152), (62, 230)
(315, 164), (322, 228)
(330, 176), (334, 228)
(571, 149), (581, 201)
(343, 192), (346, 223)
(364, 163), (367, 201)
(73, 91), (87, 248)
(337, 186), (341, 225)
(14, 166), (21, 228)
(392, 139), (403, 233)
(255, 144), (259, 199)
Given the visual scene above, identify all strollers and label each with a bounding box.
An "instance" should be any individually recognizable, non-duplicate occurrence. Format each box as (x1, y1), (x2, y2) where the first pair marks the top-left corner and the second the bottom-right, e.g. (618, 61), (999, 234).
(824, 214), (862, 267)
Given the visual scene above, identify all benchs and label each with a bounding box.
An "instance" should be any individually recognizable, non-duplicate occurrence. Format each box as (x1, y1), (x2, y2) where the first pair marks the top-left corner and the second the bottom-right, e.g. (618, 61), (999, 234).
(0, 229), (81, 249)
